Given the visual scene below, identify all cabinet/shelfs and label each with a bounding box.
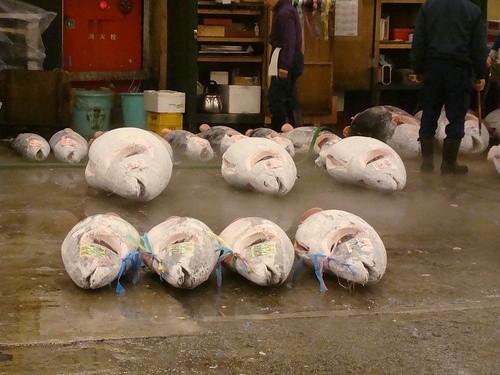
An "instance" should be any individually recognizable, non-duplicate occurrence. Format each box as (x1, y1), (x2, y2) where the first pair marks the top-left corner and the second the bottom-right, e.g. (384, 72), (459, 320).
(167, 0), (272, 127)
(373, 0), (427, 106)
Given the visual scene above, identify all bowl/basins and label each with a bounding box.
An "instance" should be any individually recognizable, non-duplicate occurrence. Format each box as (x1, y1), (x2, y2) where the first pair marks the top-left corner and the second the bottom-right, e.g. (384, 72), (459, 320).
(390, 28), (415, 41)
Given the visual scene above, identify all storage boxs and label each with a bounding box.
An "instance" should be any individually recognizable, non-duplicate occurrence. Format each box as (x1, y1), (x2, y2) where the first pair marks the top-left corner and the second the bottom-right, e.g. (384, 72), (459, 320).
(146, 123), (183, 137)
(197, 25), (225, 37)
(146, 111), (183, 126)
(202, 18), (233, 26)
(209, 70), (229, 85)
(143, 89), (186, 114)
(229, 73), (261, 85)
(390, 28), (414, 41)
(211, 85), (261, 114)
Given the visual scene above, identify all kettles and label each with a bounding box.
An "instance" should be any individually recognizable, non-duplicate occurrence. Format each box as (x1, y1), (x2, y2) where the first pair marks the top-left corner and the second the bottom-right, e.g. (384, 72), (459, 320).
(201, 80), (223, 114)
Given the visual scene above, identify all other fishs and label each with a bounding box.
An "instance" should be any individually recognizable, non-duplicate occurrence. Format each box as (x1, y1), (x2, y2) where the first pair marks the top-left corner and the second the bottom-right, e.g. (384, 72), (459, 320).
(342, 102), (500, 176)
(159, 122), (343, 164)
(84, 126), (173, 202)
(220, 136), (298, 197)
(318, 135), (407, 194)
(60, 205), (388, 296)
(10, 127), (89, 164)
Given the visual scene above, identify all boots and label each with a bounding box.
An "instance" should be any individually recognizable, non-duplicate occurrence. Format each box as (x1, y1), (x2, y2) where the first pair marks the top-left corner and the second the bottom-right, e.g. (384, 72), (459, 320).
(421, 140), (434, 171)
(441, 138), (468, 175)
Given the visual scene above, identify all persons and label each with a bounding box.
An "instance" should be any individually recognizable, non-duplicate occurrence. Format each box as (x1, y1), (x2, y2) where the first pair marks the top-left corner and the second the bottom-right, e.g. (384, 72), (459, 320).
(486, 34), (500, 67)
(268, 0), (304, 129)
(411, 0), (487, 174)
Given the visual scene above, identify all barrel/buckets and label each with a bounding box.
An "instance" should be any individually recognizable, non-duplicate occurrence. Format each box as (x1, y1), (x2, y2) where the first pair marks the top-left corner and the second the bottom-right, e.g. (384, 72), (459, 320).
(70, 89), (115, 141)
(120, 92), (147, 130)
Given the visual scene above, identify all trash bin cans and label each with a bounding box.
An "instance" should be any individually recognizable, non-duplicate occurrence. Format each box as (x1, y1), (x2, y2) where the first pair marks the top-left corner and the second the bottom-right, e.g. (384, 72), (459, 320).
(120, 92), (148, 130)
(71, 91), (114, 142)
